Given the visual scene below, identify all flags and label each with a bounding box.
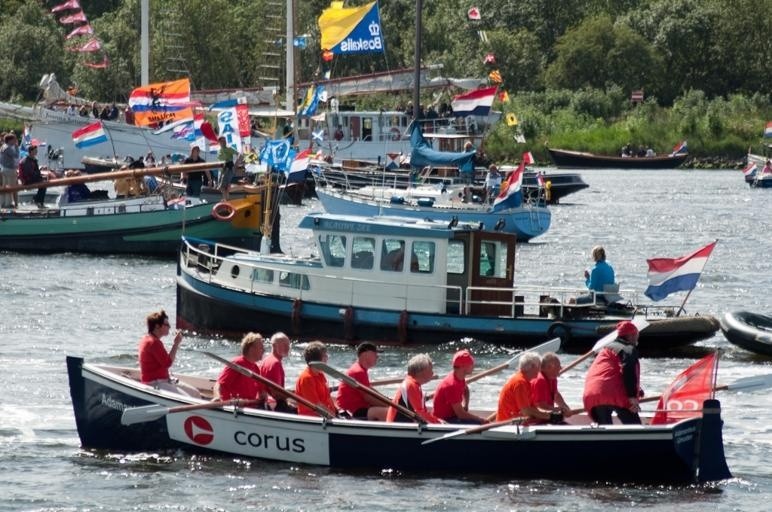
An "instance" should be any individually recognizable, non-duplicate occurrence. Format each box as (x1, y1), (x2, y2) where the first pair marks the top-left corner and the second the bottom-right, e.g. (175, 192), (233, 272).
(68, 121), (110, 150)
(672, 140), (688, 154)
(124, 76), (344, 182)
(450, 7), (546, 213)
(741, 162), (757, 175)
(645, 241), (716, 302)
(763, 122), (772, 139)
(650, 350), (717, 425)
(317, 1), (384, 54)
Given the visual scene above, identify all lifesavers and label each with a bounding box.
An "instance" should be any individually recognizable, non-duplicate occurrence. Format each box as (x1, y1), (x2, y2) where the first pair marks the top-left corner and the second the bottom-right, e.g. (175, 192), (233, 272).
(212, 202), (237, 221)
(547, 321), (571, 346)
(389, 127), (400, 141)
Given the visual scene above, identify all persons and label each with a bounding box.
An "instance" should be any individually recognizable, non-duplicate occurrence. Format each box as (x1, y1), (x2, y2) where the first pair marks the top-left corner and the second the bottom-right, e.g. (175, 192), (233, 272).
(66, 95), (135, 126)
(583, 321), (644, 424)
(570, 246), (614, 304)
(139, 310), (200, 399)
(1, 130), (246, 209)
(324, 98), (507, 203)
(762, 159), (772, 175)
(387, 241), (419, 272)
(209, 332), (573, 425)
(620, 143), (656, 158)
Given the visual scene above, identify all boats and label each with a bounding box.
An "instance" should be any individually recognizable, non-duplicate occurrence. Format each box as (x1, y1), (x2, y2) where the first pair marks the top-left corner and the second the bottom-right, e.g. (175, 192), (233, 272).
(64, 355), (732, 485)
(547, 148), (688, 169)
(418, 117), (483, 151)
(175, 213), (719, 346)
(0, 110), (476, 172)
(307, 165), (588, 201)
(719, 306), (771, 354)
(758, 172), (772, 188)
(0, 183), (285, 255)
(312, 183), (552, 242)
(248, 158), (310, 205)
(746, 154), (771, 169)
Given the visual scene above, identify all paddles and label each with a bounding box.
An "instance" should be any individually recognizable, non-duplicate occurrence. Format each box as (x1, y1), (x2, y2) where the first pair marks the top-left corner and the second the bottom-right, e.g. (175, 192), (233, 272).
(421, 409), (586, 444)
(426, 338), (561, 400)
(638, 380), (766, 402)
(559, 316), (650, 375)
(309, 362), (427, 423)
(121, 390), (297, 425)
(202, 351), (335, 418)
(329, 372), (483, 392)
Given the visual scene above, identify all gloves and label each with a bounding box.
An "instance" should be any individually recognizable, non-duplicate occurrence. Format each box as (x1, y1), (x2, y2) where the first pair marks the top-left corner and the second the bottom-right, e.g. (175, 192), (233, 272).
(549, 411), (563, 424)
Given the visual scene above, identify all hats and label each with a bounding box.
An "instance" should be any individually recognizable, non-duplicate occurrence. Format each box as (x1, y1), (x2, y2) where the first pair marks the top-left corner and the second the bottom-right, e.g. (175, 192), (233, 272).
(358, 341), (384, 353)
(452, 350), (474, 367)
(616, 321), (639, 337)
